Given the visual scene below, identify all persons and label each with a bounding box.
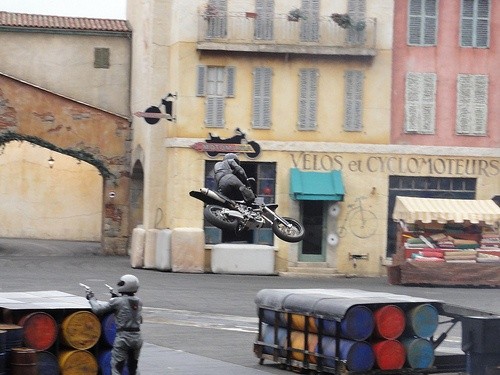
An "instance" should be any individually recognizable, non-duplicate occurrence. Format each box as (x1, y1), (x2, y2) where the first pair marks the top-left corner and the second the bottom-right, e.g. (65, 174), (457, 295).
(81, 275), (143, 375)
(213, 153), (256, 202)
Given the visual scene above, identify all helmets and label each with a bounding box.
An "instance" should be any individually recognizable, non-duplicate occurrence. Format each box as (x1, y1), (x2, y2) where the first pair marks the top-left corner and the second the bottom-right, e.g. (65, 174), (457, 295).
(223, 152), (240, 162)
(116, 274), (139, 293)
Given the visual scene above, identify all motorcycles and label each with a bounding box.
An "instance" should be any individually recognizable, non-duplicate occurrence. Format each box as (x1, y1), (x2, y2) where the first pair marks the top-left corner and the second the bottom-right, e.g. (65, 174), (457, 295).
(188, 178), (307, 243)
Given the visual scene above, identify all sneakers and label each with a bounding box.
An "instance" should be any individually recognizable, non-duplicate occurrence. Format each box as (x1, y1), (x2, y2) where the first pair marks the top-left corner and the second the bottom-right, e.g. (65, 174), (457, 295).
(240, 186), (253, 200)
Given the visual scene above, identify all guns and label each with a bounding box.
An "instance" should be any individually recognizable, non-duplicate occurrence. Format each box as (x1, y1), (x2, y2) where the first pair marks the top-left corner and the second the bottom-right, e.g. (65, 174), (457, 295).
(79, 282), (90, 294)
(105, 284), (113, 292)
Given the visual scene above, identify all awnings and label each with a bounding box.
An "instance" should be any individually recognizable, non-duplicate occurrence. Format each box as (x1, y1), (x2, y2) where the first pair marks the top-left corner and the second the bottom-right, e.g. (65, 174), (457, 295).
(292, 169), (345, 203)
(391, 195), (500, 230)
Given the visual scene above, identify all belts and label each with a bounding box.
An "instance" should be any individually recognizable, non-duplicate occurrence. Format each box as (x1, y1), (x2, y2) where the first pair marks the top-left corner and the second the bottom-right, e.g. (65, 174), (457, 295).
(116, 326), (141, 333)
